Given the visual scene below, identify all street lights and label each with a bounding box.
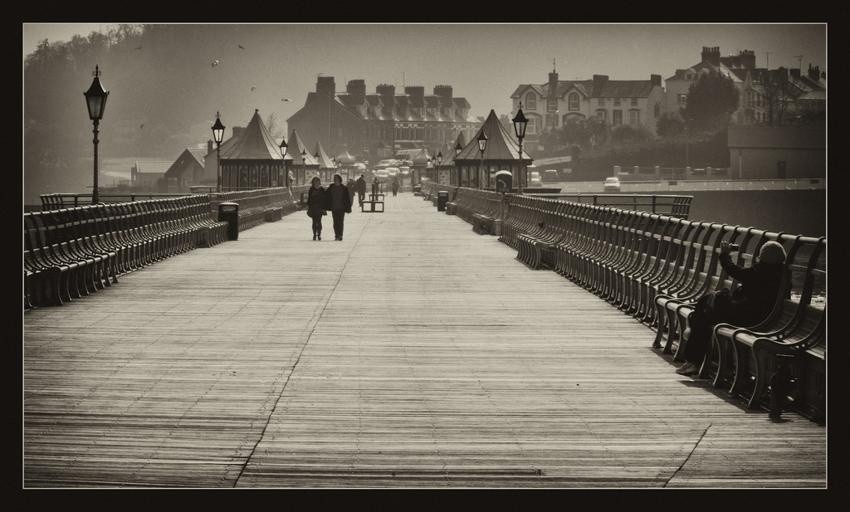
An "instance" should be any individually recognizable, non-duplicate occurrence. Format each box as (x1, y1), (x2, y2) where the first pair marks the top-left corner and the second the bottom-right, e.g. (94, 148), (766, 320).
(476, 127), (488, 190)
(511, 99), (529, 192)
(279, 135), (320, 189)
(427, 142), (464, 187)
(210, 111), (228, 192)
(83, 59), (109, 203)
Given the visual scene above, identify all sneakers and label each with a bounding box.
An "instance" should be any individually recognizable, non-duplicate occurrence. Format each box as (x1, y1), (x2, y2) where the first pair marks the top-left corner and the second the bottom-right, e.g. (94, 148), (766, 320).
(313, 233), (342, 241)
(675, 362), (697, 375)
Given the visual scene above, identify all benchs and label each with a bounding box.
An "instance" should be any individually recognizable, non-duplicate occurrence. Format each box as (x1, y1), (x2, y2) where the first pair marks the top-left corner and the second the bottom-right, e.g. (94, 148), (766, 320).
(24, 236), (119, 308)
(194, 213), (229, 247)
(263, 206), (283, 222)
(515, 226), (565, 269)
(652, 277), (825, 410)
(471, 209), (499, 235)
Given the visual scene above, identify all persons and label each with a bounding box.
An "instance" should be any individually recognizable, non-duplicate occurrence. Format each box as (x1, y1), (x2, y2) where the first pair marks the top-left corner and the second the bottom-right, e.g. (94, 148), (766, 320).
(371, 177), (379, 201)
(392, 177), (400, 197)
(305, 174), (366, 241)
(675, 238), (792, 375)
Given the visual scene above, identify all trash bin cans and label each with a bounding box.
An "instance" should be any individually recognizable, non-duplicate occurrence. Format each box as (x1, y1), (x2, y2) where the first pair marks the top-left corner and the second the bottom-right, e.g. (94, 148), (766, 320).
(438, 191), (449, 211)
(669, 180), (676, 185)
(811, 180), (820, 183)
(218, 202), (239, 241)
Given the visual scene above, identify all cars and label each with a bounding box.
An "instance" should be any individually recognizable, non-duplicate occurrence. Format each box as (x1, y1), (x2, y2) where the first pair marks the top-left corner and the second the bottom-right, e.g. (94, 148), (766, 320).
(603, 175), (620, 192)
(373, 166), (411, 184)
